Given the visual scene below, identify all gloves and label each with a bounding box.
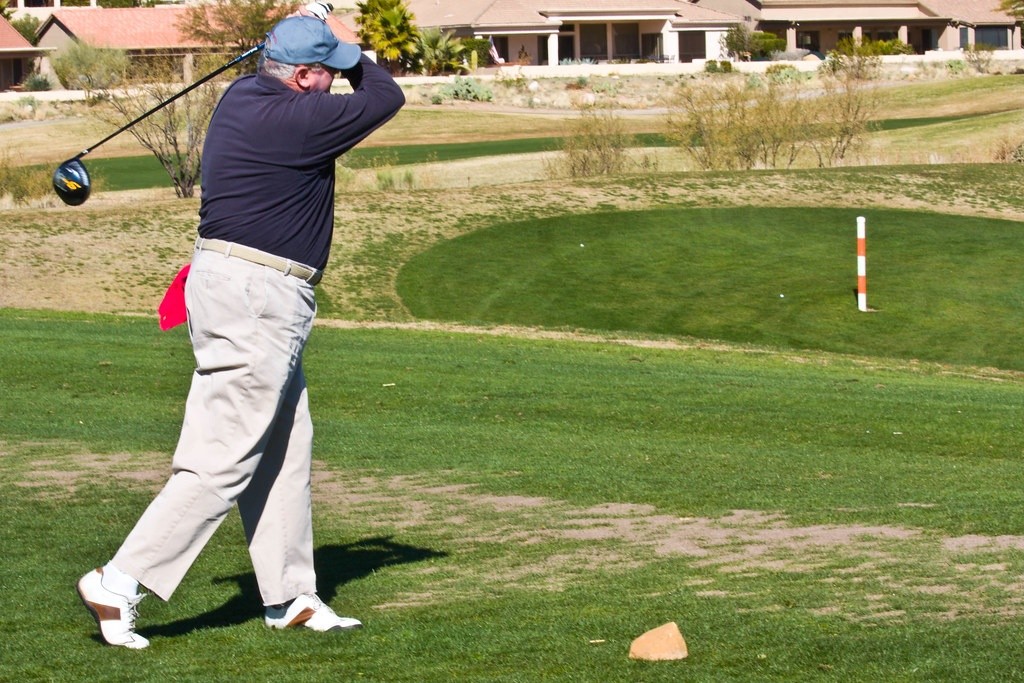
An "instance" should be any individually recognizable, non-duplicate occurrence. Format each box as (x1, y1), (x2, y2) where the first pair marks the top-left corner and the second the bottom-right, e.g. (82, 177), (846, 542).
(305, 2), (332, 20)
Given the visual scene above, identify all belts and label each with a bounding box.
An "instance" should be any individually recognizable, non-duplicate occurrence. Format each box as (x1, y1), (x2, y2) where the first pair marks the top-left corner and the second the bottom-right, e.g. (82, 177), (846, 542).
(196, 237), (323, 286)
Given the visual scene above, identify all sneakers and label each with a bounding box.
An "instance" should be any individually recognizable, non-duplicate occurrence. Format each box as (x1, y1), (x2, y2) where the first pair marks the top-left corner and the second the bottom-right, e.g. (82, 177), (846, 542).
(76, 566), (150, 649)
(264, 593), (363, 632)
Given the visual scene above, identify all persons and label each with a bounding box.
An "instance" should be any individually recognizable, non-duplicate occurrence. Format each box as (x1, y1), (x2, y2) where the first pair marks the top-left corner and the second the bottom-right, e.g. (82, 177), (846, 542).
(77, 5), (406, 649)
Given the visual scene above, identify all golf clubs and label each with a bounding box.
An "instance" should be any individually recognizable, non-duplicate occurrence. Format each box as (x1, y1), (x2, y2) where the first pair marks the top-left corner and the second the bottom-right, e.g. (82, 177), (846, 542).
(54, 1), (335, 208)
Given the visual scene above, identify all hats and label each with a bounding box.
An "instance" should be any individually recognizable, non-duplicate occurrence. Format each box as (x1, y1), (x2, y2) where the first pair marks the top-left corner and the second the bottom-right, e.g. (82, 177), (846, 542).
(263, 16), (361, 69)
